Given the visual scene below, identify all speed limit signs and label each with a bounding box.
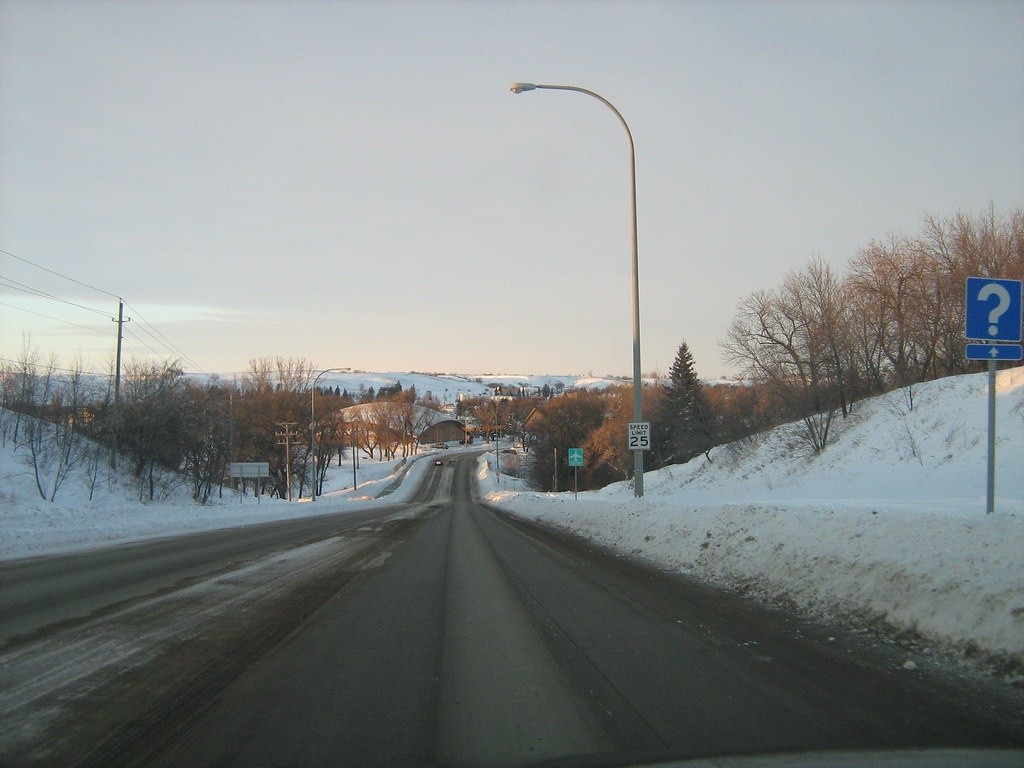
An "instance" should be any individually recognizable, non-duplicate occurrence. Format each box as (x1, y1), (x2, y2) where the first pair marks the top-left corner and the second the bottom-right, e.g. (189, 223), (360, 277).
(627, 422), (651, 451)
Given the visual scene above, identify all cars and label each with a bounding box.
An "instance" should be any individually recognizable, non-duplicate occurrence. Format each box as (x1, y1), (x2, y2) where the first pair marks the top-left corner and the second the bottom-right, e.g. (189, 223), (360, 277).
(435, 460), (443, 465)
(435, 443), (449, 449)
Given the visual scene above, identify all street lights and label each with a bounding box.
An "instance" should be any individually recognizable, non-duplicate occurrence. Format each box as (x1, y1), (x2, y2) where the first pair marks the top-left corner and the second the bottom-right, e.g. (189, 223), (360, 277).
(465, 405), (501, 484)
(311, 368), (351, 502)
(509, 82), (645, 497)
